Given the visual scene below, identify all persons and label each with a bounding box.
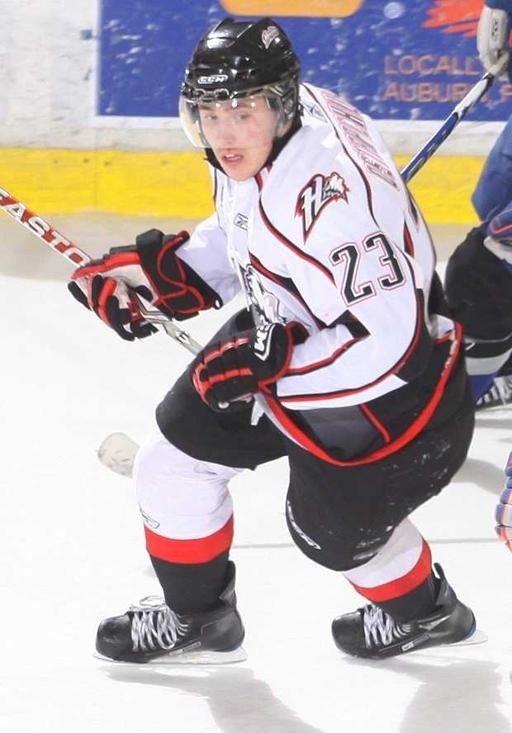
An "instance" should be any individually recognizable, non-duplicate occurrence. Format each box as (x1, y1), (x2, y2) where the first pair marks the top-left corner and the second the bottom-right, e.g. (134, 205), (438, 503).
(444, 1), (511, 553)
(67, 16), (476, 664)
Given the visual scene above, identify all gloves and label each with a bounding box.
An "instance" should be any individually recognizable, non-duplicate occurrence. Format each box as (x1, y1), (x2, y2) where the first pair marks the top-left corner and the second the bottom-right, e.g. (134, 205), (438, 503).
(68, 229), (225, 343)
(192, 322), (309, 414)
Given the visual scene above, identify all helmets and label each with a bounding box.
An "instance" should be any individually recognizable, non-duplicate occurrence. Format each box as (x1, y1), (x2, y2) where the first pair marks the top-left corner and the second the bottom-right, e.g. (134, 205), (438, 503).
(179, 18), (299, 150)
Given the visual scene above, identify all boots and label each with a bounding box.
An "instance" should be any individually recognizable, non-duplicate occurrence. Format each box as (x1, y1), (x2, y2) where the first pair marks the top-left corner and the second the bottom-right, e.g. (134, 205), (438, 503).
(332, 563), (476, 659)
(96, 562), (245, 662)
